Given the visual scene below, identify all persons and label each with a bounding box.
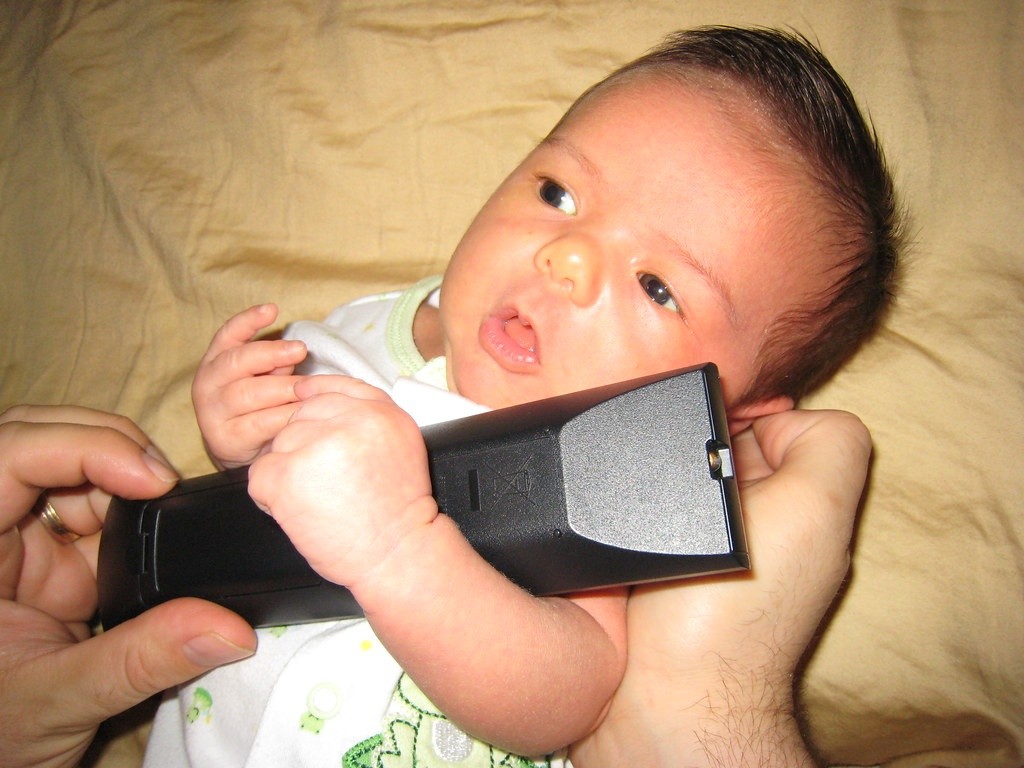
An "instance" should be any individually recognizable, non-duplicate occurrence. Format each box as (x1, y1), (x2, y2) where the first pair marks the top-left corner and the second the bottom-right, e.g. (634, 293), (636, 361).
(1, 404), (873, 768)
(144, 23), (926, 768)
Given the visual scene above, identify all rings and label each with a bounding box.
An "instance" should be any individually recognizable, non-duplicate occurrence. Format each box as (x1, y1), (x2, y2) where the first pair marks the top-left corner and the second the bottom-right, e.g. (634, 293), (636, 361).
(32, 496), (81, 546)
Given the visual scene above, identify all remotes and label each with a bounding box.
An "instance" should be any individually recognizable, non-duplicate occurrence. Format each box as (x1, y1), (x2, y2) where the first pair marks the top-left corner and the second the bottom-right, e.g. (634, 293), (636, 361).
(98, 364), (743, 633)
